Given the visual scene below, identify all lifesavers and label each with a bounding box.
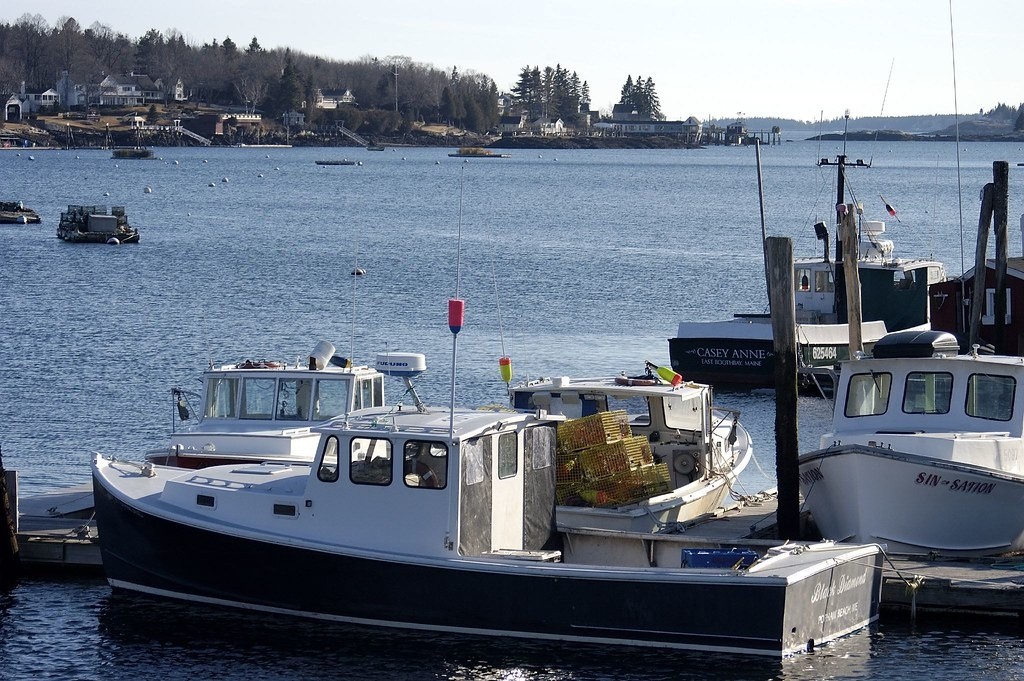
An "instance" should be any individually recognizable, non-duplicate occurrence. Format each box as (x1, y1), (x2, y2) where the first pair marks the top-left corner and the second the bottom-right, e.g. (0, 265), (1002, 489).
(614, 375), (657, 386)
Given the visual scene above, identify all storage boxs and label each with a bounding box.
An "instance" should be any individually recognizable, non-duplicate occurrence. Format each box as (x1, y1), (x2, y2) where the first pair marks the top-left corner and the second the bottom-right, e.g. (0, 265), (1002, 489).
(556, 409), (673, 509)
(681, 546), (758, 567)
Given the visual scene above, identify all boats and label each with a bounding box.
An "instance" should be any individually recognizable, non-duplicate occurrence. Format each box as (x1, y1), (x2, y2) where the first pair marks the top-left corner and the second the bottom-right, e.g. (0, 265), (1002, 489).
(794, 164), (1024, 622)
(666, 51), (948, 388)
(94, 238), (888, 655)
(479, 226), (753, 531)
(146, 338), (385, 465)
